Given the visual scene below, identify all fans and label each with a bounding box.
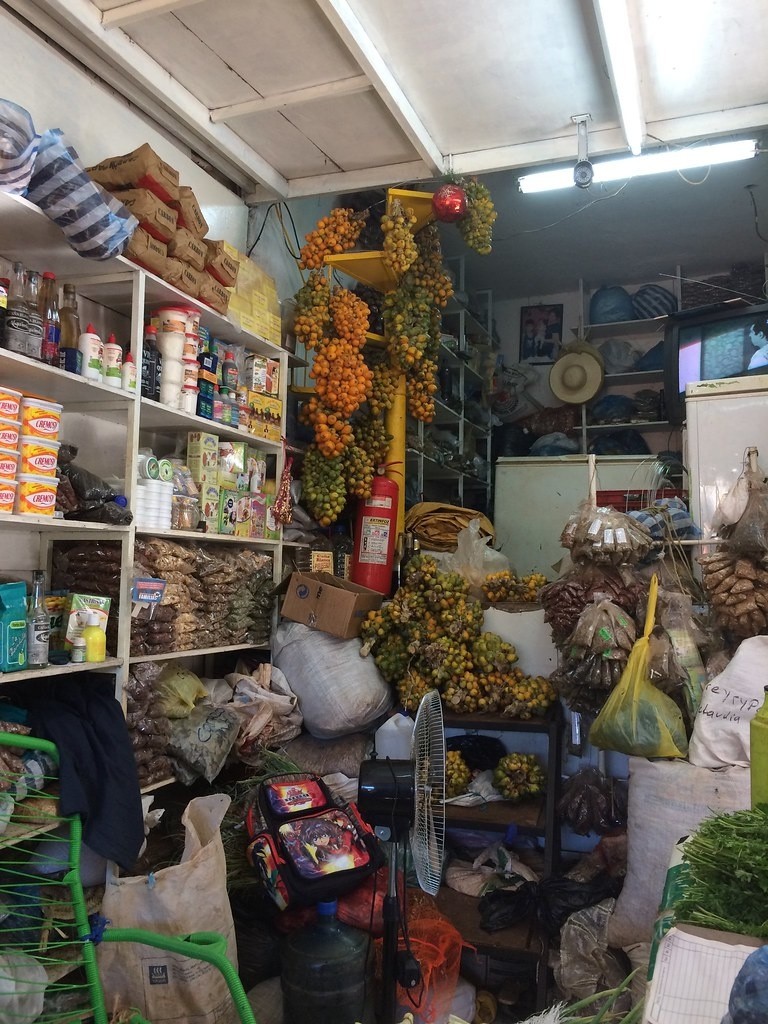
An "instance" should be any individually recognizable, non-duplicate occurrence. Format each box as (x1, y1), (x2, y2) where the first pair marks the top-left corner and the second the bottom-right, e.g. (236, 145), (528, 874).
(358, 686), (446, 1024)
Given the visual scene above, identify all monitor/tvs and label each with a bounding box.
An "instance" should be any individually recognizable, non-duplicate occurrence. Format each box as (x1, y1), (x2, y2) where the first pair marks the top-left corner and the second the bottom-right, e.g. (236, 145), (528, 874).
(663, 303), (768, 425)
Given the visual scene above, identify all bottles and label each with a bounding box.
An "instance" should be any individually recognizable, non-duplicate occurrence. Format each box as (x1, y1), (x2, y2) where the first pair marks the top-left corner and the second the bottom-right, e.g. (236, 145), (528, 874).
(72, 638), (85, 662)
(82, 614), (106, 663)
(282, 895), (375, 1024)
(0, 258), (161, 401)
(212, 352), (239, 428)
(27, 573), (49, 670)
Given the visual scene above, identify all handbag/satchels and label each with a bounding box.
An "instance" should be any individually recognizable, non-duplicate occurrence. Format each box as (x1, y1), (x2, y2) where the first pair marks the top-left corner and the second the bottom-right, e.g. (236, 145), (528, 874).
(589, 572), (690, 758)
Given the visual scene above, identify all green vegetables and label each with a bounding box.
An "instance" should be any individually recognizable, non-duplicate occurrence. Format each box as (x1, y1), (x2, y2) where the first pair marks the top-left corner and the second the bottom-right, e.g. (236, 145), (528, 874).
(675, 803), (767, 941)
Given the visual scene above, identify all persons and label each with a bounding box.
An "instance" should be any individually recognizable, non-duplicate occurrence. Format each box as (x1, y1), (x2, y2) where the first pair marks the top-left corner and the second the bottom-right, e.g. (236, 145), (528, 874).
(748, 321), (768, 370)
(523, 307), (560, 362)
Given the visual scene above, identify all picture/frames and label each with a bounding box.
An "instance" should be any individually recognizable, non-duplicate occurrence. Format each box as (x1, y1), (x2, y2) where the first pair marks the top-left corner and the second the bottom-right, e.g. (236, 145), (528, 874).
(518, 303), (563, 365)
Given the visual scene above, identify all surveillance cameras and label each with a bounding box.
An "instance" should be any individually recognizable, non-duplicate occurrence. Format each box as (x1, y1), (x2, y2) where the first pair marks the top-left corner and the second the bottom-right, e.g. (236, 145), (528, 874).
(573, 160), (594, 188)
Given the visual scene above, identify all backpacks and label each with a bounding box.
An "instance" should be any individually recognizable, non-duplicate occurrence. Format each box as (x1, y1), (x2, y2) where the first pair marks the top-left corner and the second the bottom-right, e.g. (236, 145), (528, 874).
(244, 771), (387, 912)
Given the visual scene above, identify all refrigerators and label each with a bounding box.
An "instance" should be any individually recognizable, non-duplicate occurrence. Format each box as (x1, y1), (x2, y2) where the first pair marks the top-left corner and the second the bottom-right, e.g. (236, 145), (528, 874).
(684, 373), (768, 581)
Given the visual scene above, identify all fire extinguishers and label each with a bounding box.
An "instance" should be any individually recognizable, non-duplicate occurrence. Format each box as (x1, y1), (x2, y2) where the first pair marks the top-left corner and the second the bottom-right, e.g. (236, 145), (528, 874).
(351, 461), (404, 597)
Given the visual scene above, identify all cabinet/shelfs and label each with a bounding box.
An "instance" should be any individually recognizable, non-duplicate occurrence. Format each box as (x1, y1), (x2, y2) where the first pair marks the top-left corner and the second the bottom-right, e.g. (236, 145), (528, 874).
(398, 249), (495, 514)
(390, 705), (561, 885)
(1, 187), (311, 857)
(573, 263), (683, 458)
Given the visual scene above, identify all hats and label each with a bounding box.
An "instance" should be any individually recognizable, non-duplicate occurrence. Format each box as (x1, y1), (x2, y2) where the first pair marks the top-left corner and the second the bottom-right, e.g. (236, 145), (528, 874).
(549, 350), (604, 404)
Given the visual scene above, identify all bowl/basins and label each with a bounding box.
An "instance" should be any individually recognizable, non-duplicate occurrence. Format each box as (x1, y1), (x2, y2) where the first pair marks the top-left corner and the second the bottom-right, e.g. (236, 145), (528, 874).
(155, 304), (201, 415)
(0, 387), (64, 519)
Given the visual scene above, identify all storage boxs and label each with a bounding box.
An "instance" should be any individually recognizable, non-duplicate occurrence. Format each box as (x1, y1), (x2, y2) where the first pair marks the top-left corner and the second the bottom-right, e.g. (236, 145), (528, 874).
(283, 570), (385, 637)
(187, 240), (283, 540)
(60, 593), (112, 649)
